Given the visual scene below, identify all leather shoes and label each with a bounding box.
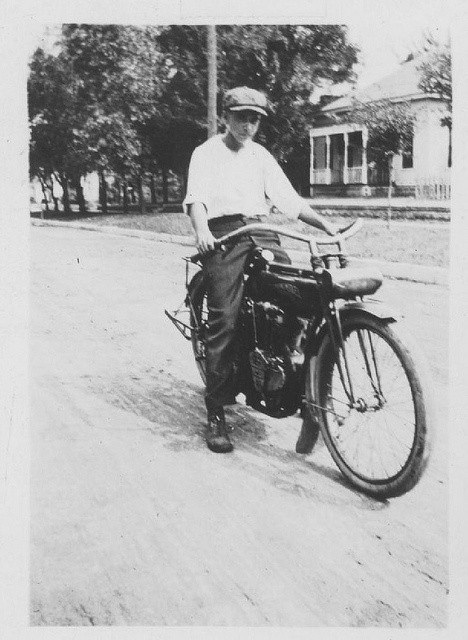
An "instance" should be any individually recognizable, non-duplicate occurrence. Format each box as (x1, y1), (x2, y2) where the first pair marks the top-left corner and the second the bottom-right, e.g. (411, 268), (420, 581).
(206, 412), (233, 453)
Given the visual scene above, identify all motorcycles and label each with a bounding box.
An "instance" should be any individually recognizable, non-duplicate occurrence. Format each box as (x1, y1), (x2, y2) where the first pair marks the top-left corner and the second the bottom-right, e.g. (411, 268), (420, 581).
(164, 217), (435, 499)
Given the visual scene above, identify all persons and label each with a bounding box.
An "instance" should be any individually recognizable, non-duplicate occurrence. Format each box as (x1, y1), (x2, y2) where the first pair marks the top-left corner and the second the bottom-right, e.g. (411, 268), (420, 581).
(182, 86), (344, 451)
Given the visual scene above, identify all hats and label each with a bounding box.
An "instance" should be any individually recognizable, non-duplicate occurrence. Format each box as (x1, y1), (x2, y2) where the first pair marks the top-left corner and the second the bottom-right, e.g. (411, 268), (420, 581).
(222, 86), (268, 118)
